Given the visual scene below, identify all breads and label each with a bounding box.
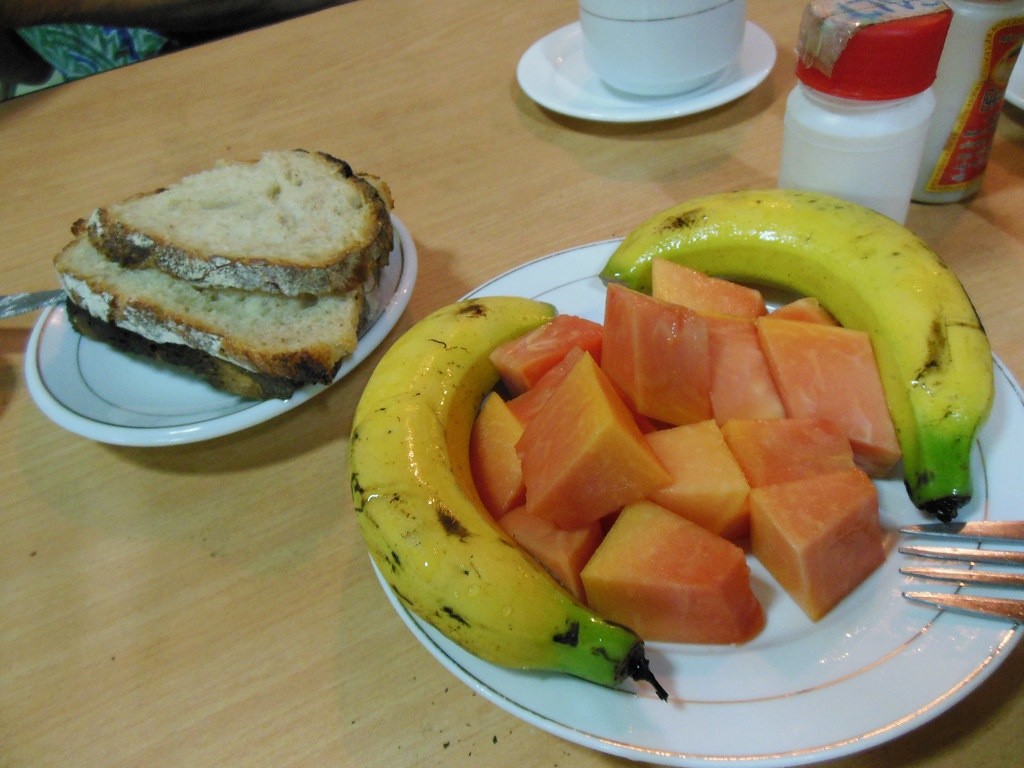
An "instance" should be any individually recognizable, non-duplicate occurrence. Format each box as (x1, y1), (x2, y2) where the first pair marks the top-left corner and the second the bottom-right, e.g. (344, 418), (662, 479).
(52, 148), (394, 402)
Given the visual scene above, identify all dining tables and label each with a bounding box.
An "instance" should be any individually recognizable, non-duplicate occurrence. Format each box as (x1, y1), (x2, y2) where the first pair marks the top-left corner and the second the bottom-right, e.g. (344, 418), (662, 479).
(0, 1), (1024, 768)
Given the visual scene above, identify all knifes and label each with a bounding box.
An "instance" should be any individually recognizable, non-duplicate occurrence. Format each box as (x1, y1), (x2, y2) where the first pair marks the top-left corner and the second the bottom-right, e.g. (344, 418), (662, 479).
(0, 289), (65, 327)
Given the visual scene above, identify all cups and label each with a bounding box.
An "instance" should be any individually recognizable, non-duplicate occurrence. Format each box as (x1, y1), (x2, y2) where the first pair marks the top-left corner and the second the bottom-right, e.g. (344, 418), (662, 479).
(577, 0), (745, 100)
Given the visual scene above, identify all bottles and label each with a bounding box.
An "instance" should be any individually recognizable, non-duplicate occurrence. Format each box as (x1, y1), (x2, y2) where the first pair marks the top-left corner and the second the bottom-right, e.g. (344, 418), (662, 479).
(904, 1), (1024, 205)
(776, 0), (953, 243)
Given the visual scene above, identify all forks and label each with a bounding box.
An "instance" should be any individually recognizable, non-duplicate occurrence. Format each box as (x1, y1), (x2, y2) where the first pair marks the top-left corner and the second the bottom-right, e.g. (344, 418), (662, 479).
(893, 518), (1024, 627)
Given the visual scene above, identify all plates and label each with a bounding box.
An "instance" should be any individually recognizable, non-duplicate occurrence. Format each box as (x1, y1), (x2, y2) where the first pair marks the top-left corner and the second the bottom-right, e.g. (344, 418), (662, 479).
(352, 234), (1024, 768)
(515, 18), (776, 123)
(22, 210), (419, 445)
(1003, 44), (1024, 112)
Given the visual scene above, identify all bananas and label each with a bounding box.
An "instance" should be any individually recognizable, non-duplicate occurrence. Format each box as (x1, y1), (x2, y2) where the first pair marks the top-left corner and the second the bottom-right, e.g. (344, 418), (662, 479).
(350, 295), (668, 701)
(596, 188), (992, 519)
